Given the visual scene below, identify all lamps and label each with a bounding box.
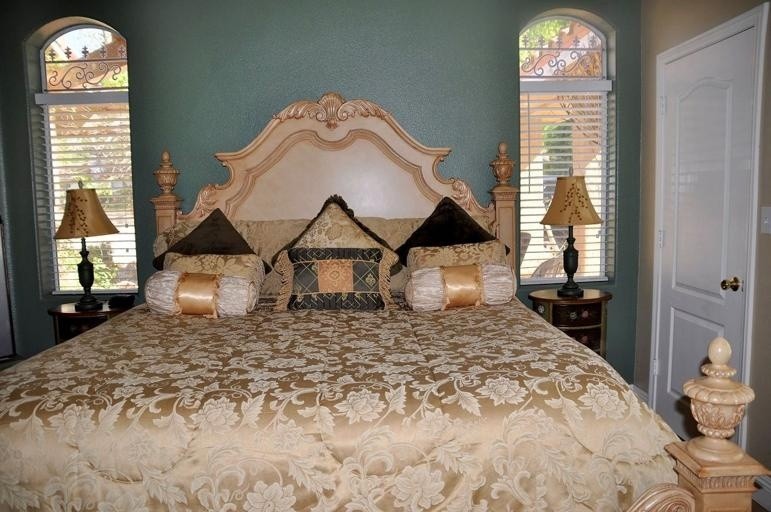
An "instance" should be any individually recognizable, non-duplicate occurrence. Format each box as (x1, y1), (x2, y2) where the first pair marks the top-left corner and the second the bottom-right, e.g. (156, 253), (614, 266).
(540, 175), (603, 297)
(54, 180), (119, 310)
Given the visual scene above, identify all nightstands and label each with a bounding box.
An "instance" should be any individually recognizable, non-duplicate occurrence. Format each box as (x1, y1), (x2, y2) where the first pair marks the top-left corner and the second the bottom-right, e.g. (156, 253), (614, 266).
(47, 301), (116, 345)
(527, 289), (612, 357)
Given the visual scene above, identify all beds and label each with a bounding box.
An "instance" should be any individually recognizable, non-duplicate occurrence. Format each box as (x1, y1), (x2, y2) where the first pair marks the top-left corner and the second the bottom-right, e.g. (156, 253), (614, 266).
(0, 89), (767, 511)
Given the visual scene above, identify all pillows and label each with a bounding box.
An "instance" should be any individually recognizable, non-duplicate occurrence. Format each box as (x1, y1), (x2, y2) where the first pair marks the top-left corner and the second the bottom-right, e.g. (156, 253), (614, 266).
(269, 196), (399, 265)
(402, 264), (517, 313)
(274, 248), (398, 310)
(392, 196), (512, 267)
(162, 251), (264, 278)
(405, 239), (510, 268)
(144, 269), (258, 320)
(153, 208), (273, 276)
